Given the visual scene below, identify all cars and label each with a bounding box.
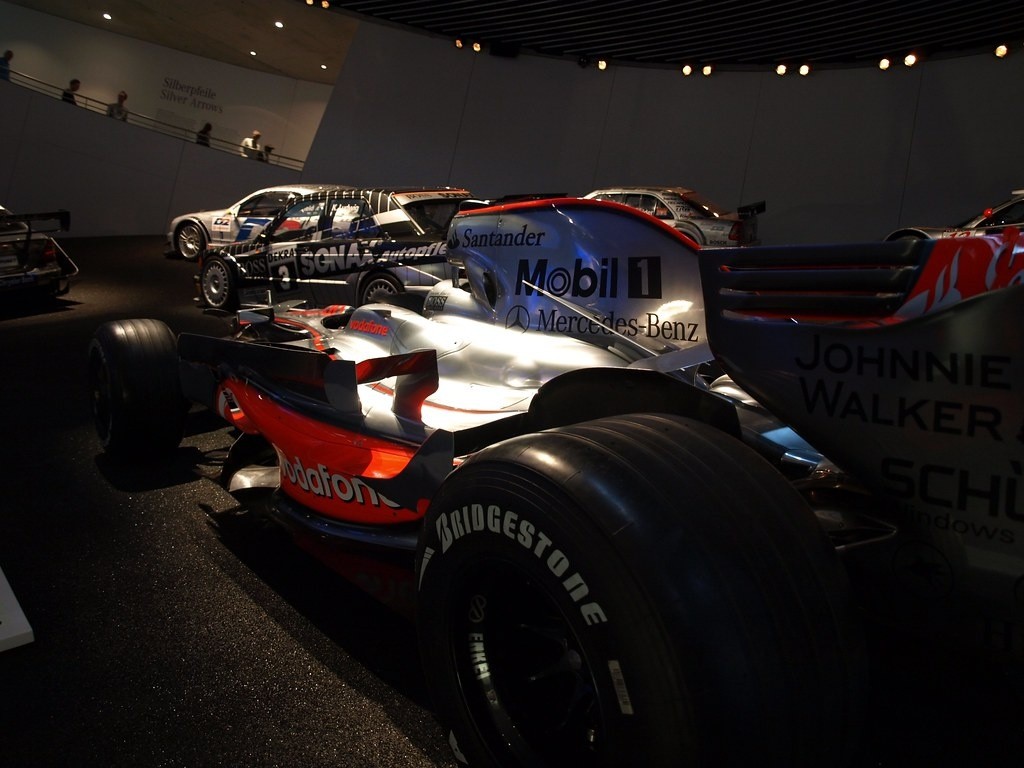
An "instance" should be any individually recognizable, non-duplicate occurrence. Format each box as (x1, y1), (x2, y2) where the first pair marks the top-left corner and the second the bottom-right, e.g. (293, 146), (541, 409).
(0, 204), (80, 306)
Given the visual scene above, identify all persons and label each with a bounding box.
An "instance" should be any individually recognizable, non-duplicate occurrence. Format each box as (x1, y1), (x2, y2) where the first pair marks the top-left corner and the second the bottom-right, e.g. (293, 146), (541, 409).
(197, 123), (211, 147)
(108, 91), (128, 122)
(239, 130), (261, 159)
(257, 144), (275, 162)
(62, 79), (80, 106)
(0, 51), (13, 78)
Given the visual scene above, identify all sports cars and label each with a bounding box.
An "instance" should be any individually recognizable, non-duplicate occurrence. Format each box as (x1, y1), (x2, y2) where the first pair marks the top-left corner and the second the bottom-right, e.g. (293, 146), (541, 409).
(161, 186), (363, 265)
(77, 186), (1024, 768)
